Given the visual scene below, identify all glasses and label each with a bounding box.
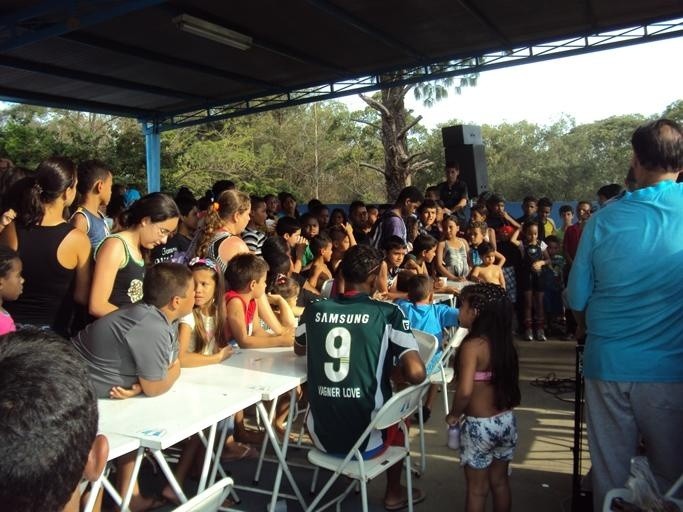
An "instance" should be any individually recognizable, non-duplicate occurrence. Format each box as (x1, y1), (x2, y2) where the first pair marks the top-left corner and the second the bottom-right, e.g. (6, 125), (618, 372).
(156, 223), (178, 237)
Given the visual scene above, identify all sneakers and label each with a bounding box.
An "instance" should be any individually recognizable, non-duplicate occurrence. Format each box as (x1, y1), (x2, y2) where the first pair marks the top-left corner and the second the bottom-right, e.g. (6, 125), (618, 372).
(414, 405), (431, 423)
(536, 329), (547, 341)
(524, 328), (533, 340)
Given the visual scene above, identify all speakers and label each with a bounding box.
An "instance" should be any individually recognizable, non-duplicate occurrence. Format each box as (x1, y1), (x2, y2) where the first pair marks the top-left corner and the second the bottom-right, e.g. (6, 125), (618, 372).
(445, 144), (489, 197)
(442, 125), (481, 147)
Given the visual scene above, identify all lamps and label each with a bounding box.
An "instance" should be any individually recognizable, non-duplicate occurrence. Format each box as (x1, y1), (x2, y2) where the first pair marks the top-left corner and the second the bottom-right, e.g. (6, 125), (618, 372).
(172, 15), (251, 52)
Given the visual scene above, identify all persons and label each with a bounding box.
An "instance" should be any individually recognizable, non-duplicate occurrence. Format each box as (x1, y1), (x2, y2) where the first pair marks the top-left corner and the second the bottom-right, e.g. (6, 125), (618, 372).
(1, 118), (682, 512)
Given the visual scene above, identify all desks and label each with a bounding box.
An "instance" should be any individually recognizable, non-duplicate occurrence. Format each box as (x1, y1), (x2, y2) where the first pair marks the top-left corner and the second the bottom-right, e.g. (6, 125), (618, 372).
(433, 290), (456, 366)
(205, 338), (308, 511)
(96, 364), (263, 512)
(80, 435), (142, 511)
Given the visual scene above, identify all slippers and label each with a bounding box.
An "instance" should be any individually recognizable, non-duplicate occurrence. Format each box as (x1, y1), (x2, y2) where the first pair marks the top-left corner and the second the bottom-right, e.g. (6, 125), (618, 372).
(141, 497), (167, 512)
(221, 442), (259, 462)
(383, 486), (426, 510)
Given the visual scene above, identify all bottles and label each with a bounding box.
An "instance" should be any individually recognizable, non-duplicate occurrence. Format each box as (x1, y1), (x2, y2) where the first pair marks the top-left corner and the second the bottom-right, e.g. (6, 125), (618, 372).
(447, 421), (459, 451)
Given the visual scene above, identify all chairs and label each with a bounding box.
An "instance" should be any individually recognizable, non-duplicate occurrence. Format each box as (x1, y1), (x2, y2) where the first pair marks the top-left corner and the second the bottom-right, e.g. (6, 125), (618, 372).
(430, 326), (468, 431)
(305, 380), (429, 511)
(169, 476), (234, 512)
(392, 328), (439, 386)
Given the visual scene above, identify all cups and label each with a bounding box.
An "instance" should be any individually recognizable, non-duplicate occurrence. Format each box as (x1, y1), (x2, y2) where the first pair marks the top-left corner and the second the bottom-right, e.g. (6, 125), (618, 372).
(438, 277), (448, 287)
(265, 218), (275, 233)
